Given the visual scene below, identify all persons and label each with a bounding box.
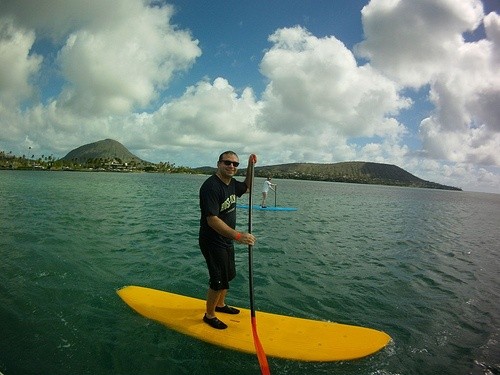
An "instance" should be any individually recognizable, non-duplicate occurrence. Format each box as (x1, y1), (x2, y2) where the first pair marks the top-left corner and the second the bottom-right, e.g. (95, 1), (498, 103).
(199, 150), (257, 329)
(260, 177), (277, 208)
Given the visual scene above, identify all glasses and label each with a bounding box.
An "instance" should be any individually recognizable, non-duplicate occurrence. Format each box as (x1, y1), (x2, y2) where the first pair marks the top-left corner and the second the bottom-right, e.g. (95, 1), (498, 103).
(220, 159), (239, 167)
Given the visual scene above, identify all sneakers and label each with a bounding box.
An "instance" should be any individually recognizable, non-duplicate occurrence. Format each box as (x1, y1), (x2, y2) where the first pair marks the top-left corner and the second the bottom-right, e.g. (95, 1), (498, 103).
(204, 314), (227, 329)
(215, 304), (240, 314)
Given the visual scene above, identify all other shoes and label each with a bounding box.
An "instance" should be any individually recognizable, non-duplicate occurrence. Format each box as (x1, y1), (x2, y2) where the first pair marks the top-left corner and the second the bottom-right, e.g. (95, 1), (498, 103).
(262, 206), (266, 208)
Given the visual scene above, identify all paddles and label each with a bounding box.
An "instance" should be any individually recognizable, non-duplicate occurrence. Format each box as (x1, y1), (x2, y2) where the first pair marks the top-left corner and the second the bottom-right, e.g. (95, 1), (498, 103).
(248, 159), (270, 375)
(274, 186), (276, 207)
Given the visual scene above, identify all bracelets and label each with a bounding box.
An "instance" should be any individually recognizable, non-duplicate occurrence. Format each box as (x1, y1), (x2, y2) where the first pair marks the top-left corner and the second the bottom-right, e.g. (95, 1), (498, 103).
(236, 232), (240, 241)
